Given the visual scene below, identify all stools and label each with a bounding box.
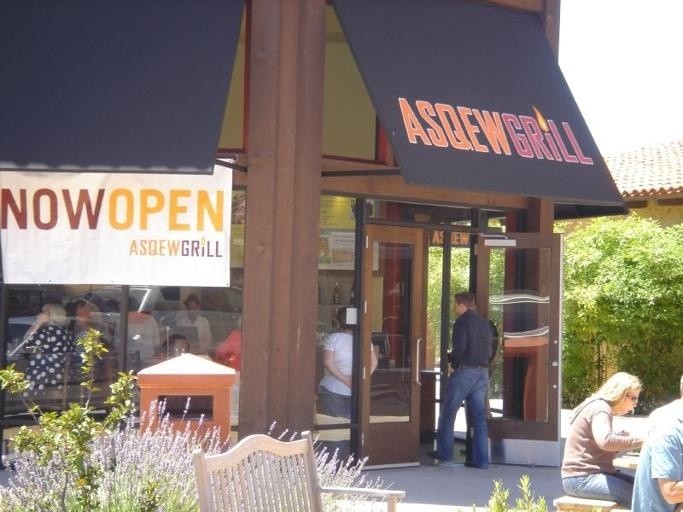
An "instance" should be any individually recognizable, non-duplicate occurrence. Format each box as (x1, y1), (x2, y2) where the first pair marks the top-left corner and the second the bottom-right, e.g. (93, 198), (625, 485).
(553, 494), (618, 512)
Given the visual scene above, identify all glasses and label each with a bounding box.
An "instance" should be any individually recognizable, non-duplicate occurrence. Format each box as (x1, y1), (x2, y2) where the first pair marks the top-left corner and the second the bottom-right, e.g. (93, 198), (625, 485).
(627, 395), (638, 402)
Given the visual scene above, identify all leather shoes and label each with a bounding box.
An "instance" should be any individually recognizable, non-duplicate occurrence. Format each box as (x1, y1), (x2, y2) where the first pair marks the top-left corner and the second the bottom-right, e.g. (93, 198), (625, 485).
(426, 451), (452, 462)
(465, 462), (488, 469)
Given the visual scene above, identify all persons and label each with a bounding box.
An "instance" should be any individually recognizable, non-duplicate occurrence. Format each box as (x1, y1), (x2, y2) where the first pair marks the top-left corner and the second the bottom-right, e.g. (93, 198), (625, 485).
(428, 290), (496, 470)
(560, 370), (642, 507)
(4, 291), (241, 411)
(318, 307), (379, 421)
(630, 374), (683, 512)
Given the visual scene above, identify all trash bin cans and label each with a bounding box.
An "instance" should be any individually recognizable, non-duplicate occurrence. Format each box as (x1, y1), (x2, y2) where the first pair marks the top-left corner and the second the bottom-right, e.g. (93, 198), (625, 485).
(137, 353), (236, 453)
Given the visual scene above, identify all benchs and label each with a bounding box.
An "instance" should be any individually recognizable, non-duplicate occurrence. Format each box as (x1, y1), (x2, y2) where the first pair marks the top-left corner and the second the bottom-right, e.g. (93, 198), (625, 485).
(193, 430), (405, 512)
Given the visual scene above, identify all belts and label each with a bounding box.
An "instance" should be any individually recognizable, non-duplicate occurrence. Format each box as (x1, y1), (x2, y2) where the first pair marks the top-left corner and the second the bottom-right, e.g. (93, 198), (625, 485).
(454, 365), (492, 368)
